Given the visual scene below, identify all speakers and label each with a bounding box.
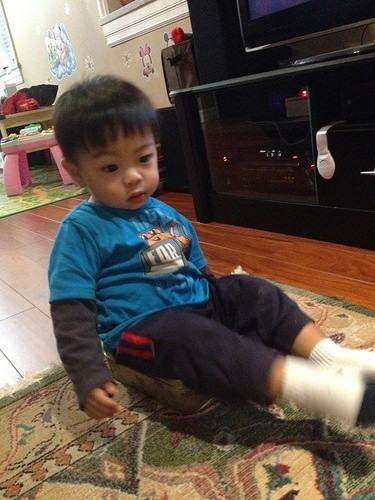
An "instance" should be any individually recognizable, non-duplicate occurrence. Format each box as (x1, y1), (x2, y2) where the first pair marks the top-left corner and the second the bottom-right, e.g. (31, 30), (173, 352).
(156, 107), (192, 194)
(187, 0), (247, 84)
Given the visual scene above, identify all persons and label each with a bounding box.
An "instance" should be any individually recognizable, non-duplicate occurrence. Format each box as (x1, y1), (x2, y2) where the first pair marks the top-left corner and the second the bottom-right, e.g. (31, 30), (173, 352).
(49, 76), (374, 428)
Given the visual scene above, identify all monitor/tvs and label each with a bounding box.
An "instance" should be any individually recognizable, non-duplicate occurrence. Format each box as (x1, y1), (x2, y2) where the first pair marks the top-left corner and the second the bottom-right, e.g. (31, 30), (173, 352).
(235, 0), (375, 73)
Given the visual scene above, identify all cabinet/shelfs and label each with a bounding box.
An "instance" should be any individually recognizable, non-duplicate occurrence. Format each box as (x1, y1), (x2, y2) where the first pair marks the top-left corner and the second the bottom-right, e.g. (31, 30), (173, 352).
(169, 50), (375, 252)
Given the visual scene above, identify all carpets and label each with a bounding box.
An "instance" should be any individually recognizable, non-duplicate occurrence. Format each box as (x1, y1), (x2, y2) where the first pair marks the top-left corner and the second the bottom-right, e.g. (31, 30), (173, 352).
(0, 266), (375, 500)
(0, 164), (89, 219)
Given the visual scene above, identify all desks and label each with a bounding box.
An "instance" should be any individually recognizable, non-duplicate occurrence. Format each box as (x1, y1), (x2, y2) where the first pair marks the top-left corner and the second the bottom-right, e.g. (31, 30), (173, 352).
(0, 104), (76, 137)
